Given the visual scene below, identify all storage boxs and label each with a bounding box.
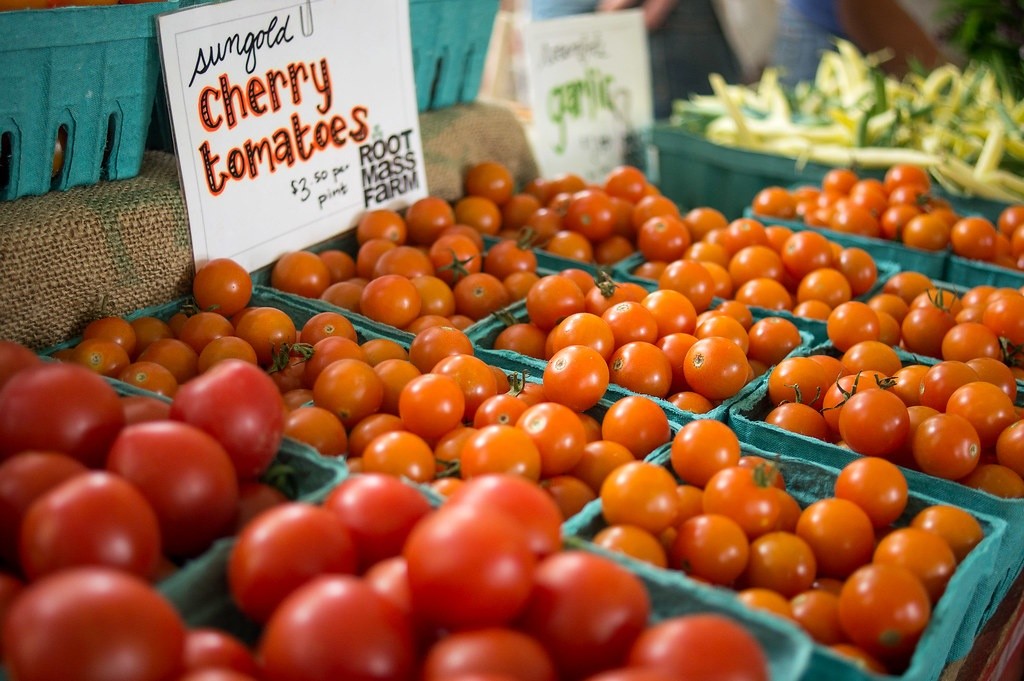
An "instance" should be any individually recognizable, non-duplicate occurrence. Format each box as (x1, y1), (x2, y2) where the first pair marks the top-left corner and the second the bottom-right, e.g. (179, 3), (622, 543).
(407, 1), (500, 114)
(32, 193), (1024, 681)
(0, 2), (179, 204)
(637, 113), (892, 220)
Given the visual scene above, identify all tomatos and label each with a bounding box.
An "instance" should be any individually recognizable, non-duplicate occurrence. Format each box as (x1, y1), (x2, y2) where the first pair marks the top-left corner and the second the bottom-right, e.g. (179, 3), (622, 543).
(0, 160), (1024, 681)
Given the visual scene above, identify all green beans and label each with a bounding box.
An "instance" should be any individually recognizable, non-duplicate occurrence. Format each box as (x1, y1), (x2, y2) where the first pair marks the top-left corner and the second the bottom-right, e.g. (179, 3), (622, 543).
(673, 36), (1024, 210)
